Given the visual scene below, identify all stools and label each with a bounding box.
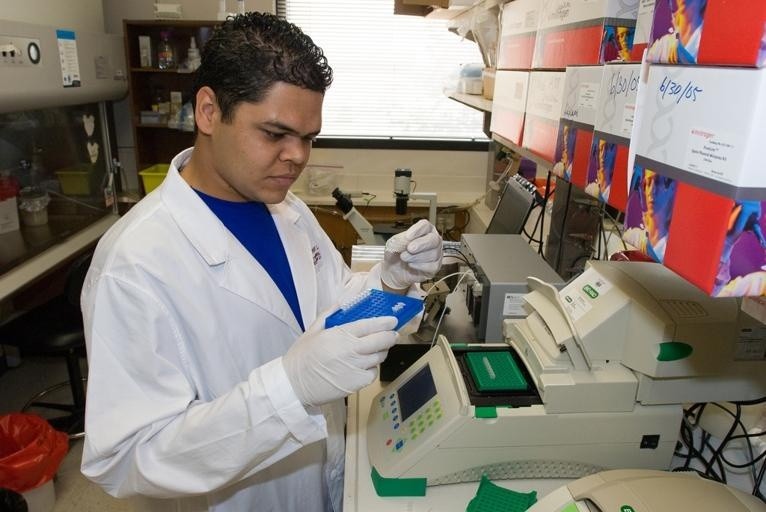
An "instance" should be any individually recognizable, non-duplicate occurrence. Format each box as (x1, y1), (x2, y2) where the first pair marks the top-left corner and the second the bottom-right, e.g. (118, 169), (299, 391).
(18, 312), (87, 441)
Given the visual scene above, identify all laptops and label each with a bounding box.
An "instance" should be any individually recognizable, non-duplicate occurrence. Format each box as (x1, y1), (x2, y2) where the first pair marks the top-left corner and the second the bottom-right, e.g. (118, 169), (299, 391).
(441, 176), (535, 258)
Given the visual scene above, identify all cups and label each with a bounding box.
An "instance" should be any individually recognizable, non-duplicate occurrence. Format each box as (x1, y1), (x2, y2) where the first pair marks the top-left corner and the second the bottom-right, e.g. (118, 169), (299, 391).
(17, 187), (48, 226)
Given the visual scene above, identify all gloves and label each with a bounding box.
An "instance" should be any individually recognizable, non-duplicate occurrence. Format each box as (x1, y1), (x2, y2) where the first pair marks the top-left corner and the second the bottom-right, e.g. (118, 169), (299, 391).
(283, 303), (399, 411)
(380, 219), (443, 290)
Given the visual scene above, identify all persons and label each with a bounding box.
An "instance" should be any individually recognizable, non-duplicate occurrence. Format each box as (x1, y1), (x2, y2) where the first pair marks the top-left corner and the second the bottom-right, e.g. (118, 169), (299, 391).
(585, 139), (616, 203)
(622, 168), (669, 264)
(79, 11), (444, 512)
(616, 27), (634, 62)
(647, 0), (706, 65)
(553, 125), (573, 181)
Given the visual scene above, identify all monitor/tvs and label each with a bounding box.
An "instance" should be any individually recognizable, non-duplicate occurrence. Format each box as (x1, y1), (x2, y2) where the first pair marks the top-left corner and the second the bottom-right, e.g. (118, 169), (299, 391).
(397, 365), (437, 422)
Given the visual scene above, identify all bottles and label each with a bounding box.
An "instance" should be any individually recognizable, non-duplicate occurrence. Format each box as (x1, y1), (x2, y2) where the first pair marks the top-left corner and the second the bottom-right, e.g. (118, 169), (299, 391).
(157, 31), (178, 69)
(187, 36), (200, 69)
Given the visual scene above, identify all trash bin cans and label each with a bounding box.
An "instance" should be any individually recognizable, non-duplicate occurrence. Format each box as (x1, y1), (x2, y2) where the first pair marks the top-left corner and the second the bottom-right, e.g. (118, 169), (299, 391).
(0, 413), (55, 512)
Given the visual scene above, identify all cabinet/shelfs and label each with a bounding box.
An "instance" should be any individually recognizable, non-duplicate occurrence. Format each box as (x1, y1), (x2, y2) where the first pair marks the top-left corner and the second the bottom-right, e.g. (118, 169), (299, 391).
(122, 18), (236, 201)
